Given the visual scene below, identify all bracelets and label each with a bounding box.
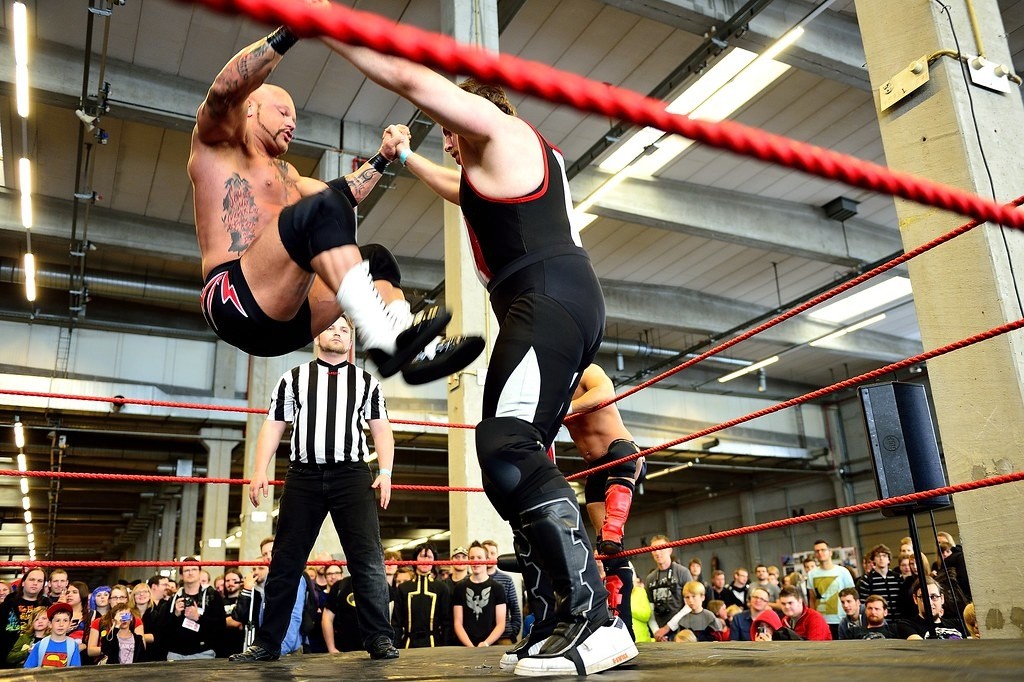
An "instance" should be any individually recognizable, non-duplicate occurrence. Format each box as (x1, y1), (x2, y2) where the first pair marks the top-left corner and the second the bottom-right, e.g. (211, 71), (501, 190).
(399, 149), (413, 168)
(380, 469), (391, 478)
(483, 640), (489, 647)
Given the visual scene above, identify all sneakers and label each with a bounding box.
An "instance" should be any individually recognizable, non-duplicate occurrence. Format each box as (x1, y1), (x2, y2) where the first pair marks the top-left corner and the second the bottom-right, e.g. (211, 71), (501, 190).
(370, 636), (399, 659)
(499, 633), (552, 669)
(401, 335), (487, 382)
(514, 615), (639, 677)
(229, 646), (281, 662)
(362, 305), (454, 380)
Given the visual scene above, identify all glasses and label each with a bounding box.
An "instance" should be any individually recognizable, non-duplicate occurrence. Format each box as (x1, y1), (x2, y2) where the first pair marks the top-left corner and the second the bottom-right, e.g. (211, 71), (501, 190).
(183, 569), (198, 574)
(916, 593), (941, 601)
(325, 572), (342, 578)
(109, 596), (127, 602)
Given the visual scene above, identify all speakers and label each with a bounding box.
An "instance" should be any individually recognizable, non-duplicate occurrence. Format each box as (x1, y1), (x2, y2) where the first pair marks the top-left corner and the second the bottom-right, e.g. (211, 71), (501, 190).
(856, 383), (950, 518)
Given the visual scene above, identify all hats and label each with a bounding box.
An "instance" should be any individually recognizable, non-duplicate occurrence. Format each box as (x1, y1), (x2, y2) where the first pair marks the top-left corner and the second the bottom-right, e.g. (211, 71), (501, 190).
(47, 601), (73, 619)
(452, 546), (468, 556)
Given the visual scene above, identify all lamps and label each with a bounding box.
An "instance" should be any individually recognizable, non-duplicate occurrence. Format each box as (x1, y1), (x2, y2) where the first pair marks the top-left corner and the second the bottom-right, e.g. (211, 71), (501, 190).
(758, 368), (766, 392)
(75, 105), (98, 132)
(617, 352), (624, 371)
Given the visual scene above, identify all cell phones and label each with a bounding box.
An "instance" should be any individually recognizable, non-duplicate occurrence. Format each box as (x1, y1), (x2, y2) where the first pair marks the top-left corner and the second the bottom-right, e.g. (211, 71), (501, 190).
(72, 619), (79, 626)
(758, 627), (766, 635)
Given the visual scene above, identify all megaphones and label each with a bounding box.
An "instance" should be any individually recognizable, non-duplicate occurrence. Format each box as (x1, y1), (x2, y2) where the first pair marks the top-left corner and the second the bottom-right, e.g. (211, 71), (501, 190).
(75, 109), (96, 132)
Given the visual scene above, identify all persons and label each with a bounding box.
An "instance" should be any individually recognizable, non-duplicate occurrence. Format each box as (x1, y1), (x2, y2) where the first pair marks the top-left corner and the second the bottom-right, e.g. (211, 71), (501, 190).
(187, 24), (486, 386)
(0, 532), (981, 667)
(315, 34), (641, 677)
(229, 314), (399, 663)
(563, 362), (647, 644)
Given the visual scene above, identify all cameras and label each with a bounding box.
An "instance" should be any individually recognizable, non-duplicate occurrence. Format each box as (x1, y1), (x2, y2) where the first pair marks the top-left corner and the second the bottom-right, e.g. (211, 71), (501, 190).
(181, 597), (194, 607)
(120, 612), (131, 621)
